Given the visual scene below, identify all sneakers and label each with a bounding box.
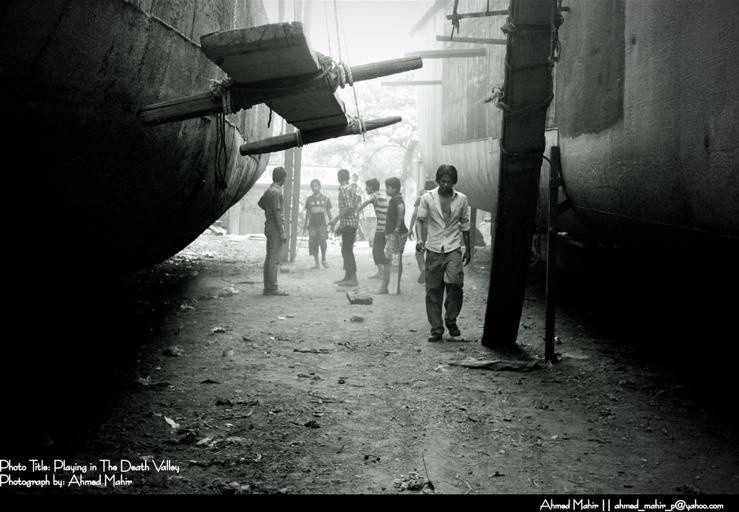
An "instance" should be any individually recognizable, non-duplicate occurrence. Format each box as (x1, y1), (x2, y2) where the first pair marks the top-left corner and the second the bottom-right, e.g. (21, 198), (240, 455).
(445, 324), (460, 336)
(428, 332), (442, 342)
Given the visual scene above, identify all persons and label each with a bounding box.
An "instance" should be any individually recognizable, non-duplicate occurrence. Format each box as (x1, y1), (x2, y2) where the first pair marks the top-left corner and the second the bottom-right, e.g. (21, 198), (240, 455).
(303, 164), (471, 342)
(257, 167), (289, 296)
